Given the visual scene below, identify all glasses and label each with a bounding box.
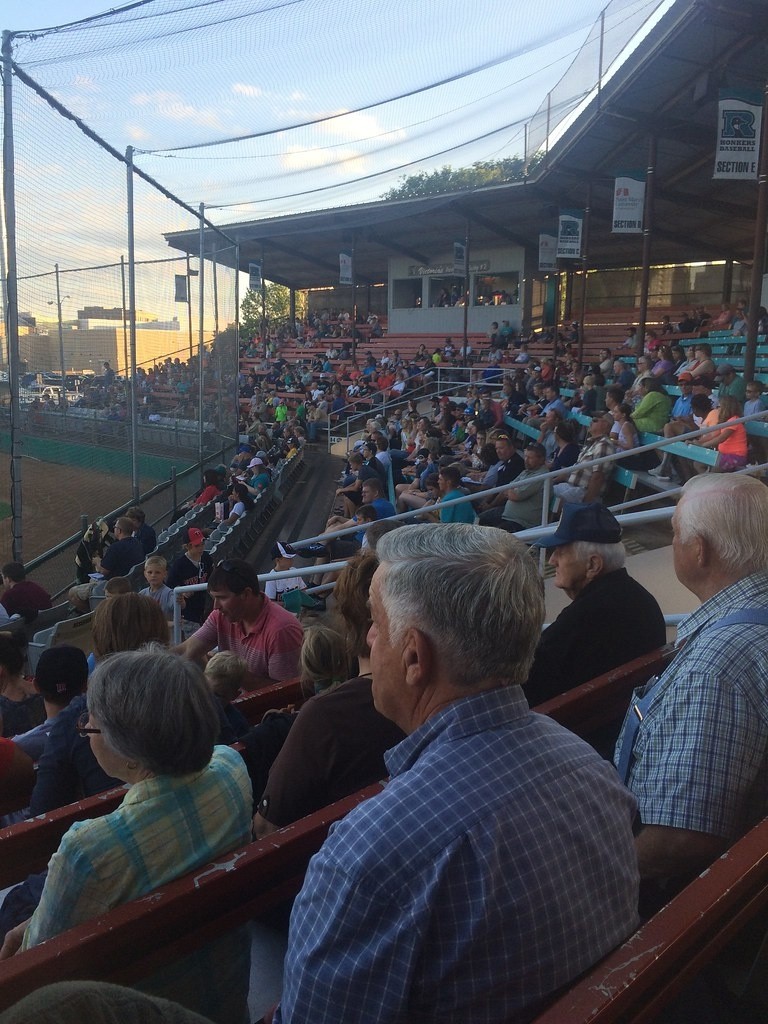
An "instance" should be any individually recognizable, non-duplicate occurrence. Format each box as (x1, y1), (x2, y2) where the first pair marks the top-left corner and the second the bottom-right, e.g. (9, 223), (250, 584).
(76, 712), (101, 738)
(746, 389), (755, 393)
(113, 525), (119, 529)
(694, 349), (701, 351)
(498, 434), (512, 446)
(592, 417), (598, 423)
(215, 559), (248, 587)
(198, 562), (205, 578)
(529, 441), (544, 450)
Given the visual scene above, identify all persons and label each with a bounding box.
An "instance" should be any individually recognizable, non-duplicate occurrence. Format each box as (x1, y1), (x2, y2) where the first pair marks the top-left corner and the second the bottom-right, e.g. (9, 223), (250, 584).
(0, 305), (473, 428)
(614, 362), (768, 488)
(612, 473), (768, 927)
(69, 508), (265, 635)
(615, 296), (768, 363)
(265, 520), (643, 1023)
(524, 499), (669, 760)
(169, 428), (308, 533)
(476, 314), (581, 346)
(0, 548), (407, 1024)
(308, 429), (615, 536)
(475, 347), (616, 428)
(409, 276), (518, 307)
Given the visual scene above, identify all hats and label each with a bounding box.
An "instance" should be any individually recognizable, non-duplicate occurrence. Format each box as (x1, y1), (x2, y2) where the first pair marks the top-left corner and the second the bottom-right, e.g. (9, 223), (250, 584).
(271, 541), (296, 560)
(247, 457), (263, 467)
(535, 504), (623, 548)
(36, 648), (89, 692)
(718, 363), (734, 381)
(255, 451), (266, 458)
(183, 527), (207, 545)
(678, 373), (692, 382)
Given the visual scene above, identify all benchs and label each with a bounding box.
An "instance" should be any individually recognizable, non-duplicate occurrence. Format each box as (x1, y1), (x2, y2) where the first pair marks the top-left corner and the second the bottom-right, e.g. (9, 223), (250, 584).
(0, 311), (768, 1024)
